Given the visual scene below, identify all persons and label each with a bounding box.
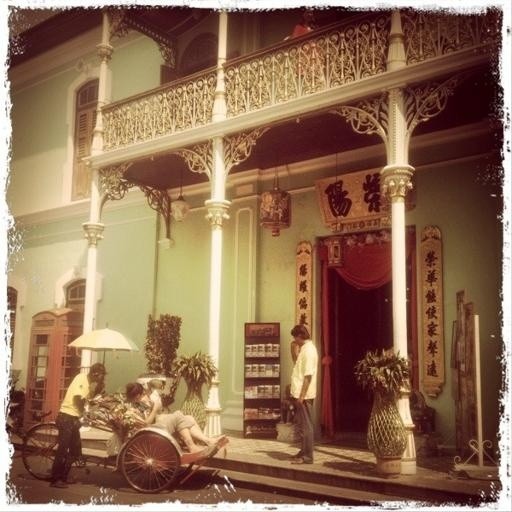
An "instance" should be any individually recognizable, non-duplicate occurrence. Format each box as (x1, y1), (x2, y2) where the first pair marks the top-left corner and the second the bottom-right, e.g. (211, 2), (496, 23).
(289, 325), (319, 465)
(122, 378), (229, 454)
(49, 362), (107, 489)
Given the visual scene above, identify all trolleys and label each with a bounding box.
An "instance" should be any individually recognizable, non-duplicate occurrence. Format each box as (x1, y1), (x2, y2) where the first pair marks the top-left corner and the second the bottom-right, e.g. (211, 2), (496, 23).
(86, 397), (228, 497)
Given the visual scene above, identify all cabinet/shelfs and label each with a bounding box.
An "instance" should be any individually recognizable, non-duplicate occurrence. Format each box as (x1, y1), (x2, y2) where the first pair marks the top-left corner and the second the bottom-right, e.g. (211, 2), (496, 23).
(243, 322), (280, 440)
(21, 307), (83, 439)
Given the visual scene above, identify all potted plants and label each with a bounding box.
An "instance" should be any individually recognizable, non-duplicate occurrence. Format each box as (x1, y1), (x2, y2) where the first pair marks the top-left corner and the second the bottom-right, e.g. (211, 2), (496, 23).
(135, 313), (188, 415)
(173, 350), (219, 434)
(353, 350), (411, 475)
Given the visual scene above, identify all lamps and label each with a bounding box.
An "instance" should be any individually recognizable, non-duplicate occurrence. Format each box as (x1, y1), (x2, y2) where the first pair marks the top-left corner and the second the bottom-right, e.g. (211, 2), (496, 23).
(258, 141), (291, 237)
(170, 162), (189, 222)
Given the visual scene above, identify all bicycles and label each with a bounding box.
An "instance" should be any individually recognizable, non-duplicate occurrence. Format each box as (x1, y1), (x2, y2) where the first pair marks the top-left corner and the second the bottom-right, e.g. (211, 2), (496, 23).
(4, 405), (70, 485)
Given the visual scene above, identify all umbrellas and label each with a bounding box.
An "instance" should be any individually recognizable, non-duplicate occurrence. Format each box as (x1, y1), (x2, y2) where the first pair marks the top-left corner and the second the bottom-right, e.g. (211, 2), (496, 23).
(67, 320), (140, 395)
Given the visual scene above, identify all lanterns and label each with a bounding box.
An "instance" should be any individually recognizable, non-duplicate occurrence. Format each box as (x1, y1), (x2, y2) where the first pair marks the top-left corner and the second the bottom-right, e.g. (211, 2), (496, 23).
(257, 172), (290, 238)
